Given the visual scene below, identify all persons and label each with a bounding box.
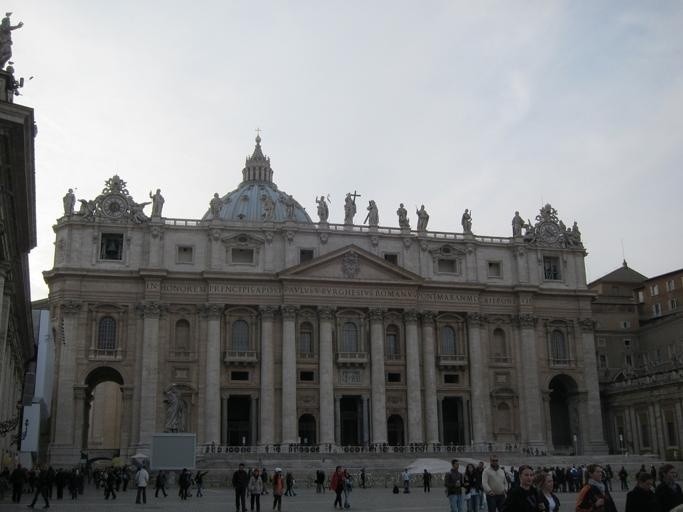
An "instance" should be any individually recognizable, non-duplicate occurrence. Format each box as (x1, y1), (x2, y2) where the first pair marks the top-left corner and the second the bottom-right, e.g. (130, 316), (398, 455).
(416, 205), (429, 231)
(345, 194), (356, 223)
(163, 382), (185, 434)
(210, 193), (222, 217)
(512, 211), (524, 238)
(231, 463), (295, 512)
(367, 200), (379, 226)
(397, 203), (410, 228)
(28, 463), (50, 509)
(315, 465), (365, 509)
(1, 462), (131, 504)
(135, 465), (149, 504)
(178, 468), (208, 500)
(150, 190), (164, 217)
(154, 469), (168, 497)
(64, 189), (76, 216)
(462, 209), (473, 233)
(404, 453), (683, 512)
(316, 196), (329, 221)
